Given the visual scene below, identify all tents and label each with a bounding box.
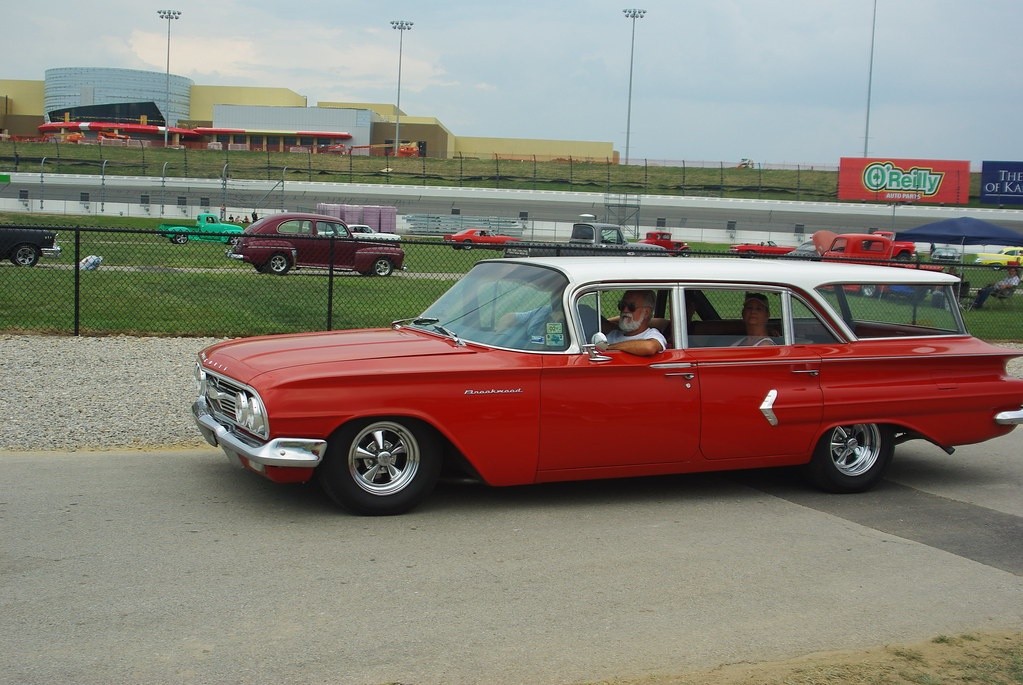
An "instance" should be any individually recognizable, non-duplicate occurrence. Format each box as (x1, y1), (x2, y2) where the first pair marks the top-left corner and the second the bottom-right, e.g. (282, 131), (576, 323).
(878, 217), (1023, 304)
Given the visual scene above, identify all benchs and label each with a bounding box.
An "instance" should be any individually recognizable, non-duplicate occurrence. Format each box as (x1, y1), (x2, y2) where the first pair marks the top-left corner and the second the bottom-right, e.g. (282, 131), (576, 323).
(847, 321), (954, 338)
(688, 320), (782, 348)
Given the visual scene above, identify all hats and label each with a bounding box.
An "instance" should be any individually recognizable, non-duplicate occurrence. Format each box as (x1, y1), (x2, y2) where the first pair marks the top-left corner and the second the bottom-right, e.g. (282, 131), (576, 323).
(743, 293), (770, 310)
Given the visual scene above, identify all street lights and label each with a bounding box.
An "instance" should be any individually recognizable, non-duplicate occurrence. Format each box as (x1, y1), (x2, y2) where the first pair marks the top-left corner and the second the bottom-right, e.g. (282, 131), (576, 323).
(623, 8), (648, 167)
(391, 20), (415, 158)
(157, 9), (182, 147)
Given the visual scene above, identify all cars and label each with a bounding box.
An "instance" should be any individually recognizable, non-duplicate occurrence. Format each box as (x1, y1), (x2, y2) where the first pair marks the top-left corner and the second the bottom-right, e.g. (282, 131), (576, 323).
(225, 212), (406, 278)
(318, 224), (401, 240)
(781, 240), (821, 262)
(638, 231), (692, 258)
(187, 254), (1023, 516)
(931, 248), (963, 264)
(975, 245), (1023, 269)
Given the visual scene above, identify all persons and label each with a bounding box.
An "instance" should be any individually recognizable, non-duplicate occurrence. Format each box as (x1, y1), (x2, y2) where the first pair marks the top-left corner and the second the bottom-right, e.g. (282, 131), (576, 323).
(606, 290), (668, 356)
(497, 285), (618, 350)
(235, 215), (242, 224)
(1016, 257), (1021, 266)
(606, 290), (781, 348)
(479, 231), (486, 236)
(932, 285), (946, 310)
(354, 227), (363, 233)
(727, 293), (777, 347)
(968, 267), (1021, 309)
(929, 239), (936, 259)
(251, 212), (258, 223)
(242, 216), (249, 223)
(219, 204), (225, 220)
(229, 214), (234, 222)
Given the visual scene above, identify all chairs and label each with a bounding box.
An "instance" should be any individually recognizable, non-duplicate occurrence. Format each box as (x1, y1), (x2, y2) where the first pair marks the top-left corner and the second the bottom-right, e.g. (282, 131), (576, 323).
(609, 318), (672, 340)
(967, 267), (1023, 311)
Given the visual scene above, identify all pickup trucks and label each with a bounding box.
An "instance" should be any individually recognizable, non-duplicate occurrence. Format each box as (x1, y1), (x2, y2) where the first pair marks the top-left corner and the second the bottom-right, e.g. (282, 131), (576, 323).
(819, 233), (945, 297)
(157, 213), (245, 245)
(0, 228), (62, 268)
(502, 222), (669, 258)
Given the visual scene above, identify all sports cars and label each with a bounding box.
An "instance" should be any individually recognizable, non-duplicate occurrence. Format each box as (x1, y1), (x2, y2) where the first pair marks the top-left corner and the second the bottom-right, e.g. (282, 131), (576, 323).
(443, 228), (522, 251)
(728, 240), (797, 259)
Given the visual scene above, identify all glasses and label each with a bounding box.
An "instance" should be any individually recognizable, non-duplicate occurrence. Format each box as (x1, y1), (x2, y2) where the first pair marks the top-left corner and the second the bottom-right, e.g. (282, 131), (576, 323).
(617, 302), (651, 312)
